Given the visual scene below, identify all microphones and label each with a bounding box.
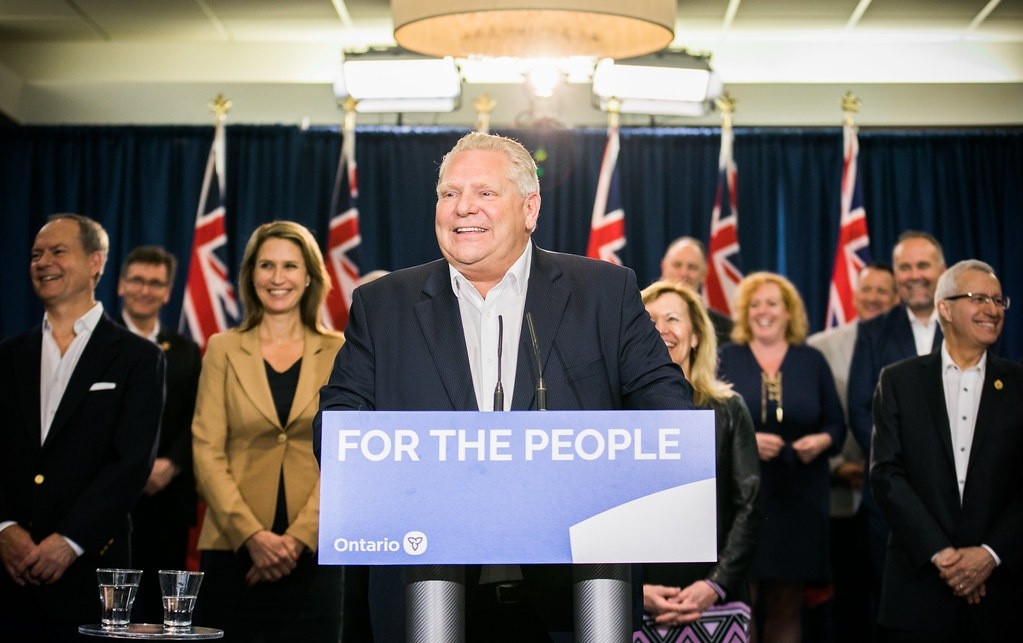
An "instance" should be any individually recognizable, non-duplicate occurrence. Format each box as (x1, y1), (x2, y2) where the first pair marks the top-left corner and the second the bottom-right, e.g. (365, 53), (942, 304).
(526, 311), (546, 411)
(493, 315), (504, 412)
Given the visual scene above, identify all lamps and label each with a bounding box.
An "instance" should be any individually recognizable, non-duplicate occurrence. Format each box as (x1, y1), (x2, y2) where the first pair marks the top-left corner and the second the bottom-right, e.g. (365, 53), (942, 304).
(391, 0), (677, 61)
(592, 53), (712, 118)
(344, 51), (463, 113)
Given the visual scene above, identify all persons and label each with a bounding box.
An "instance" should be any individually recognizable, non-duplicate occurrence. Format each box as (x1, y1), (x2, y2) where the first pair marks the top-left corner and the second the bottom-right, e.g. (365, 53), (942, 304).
(193, 223), (349, 643)
(314, 133), (696, 470)
(637, 230), (1023, 642)
(91, 244), (200, 626)
(0, 215), (164, 643)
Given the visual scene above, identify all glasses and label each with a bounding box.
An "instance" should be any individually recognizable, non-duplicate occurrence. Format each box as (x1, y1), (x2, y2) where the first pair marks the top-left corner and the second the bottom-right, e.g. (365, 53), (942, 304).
(944, 292), (1011, 311)
(122, 277), (168, 292)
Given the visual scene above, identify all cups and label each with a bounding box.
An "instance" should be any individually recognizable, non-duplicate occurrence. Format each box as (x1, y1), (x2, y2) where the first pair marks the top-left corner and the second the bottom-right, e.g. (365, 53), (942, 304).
(97, 567), (143, 633)
(158, 570), (205, 633)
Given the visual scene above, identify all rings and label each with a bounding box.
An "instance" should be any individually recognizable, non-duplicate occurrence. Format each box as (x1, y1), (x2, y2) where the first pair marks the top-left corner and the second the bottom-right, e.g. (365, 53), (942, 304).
(958, 582), (965, 591)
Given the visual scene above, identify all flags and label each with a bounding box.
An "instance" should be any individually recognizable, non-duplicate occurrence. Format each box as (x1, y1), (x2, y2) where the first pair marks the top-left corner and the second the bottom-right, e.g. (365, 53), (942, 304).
(819, 124), (872, 333)
(584, 111), (630, 266)
(308, 109), (391, 338)
(172, 123), (242, 352)
(697, 125), (743, 322)
